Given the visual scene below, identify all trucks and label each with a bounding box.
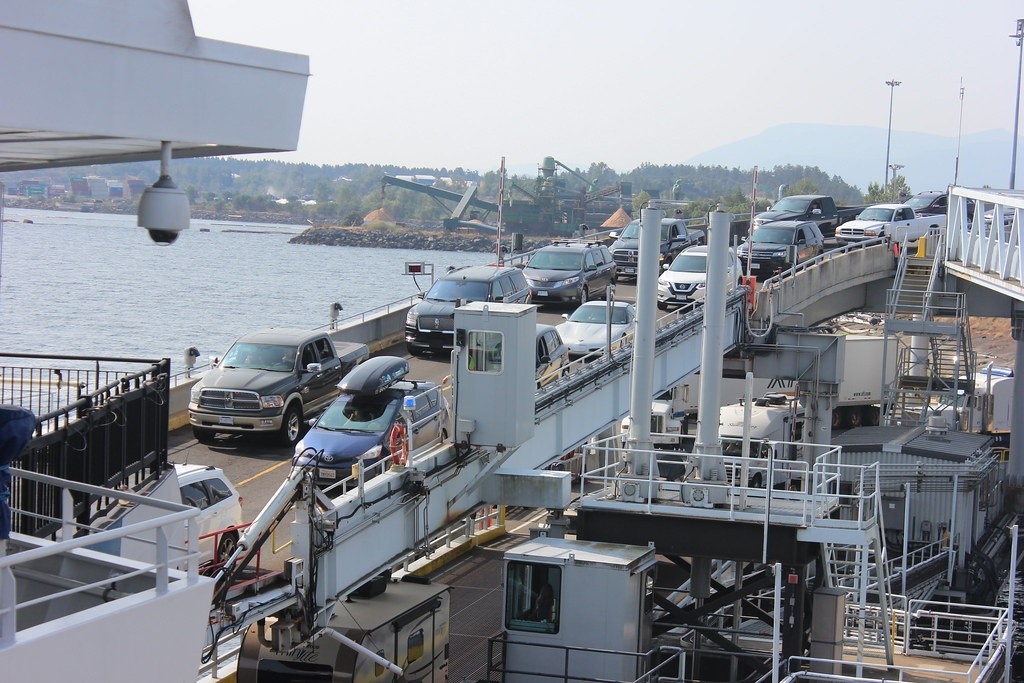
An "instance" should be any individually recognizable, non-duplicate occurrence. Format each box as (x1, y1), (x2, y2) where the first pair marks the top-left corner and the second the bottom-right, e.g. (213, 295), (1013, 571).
(718, 394), (805, 487)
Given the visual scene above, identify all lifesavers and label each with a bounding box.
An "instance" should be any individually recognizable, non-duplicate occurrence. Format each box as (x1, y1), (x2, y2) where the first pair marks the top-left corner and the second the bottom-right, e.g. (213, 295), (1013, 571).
(390, 422), (410, 466)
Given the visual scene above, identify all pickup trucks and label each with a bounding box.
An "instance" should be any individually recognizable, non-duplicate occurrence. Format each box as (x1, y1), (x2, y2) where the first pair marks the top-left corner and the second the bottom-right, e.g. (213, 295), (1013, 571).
(608, 217), (707, 282)
(187, 328), (370, 451)
(752, 195), (870, 229)
(834, 204), (948, 254)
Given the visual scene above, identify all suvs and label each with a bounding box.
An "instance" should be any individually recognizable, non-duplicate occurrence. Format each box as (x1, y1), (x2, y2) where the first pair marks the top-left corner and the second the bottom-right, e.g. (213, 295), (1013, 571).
(292, 356), (452, 491)
(522, 240), (619, 311)
(406, 265), (532, 357)
(536, 325), (570, 387)
(736, 220), (824, 281)
(904, 191), (975, 217)
(160, 452), (245, 572)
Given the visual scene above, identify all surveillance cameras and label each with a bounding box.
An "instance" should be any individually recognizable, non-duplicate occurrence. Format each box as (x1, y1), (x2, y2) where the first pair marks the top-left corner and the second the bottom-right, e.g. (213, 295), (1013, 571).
(136, 185), (191, 244)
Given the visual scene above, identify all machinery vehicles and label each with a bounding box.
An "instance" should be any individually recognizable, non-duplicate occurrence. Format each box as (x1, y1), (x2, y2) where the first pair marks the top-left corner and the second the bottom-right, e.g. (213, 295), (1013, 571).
(379, 156), (623, 230)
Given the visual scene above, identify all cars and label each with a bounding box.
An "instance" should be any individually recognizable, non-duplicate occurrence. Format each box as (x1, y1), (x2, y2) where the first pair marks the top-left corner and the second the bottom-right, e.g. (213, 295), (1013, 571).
(556, 300), (640, 356)
(656, 245), (745, 311)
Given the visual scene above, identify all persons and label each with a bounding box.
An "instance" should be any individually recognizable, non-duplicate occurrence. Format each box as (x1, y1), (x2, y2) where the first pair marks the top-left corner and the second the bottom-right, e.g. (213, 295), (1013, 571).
(283, 349), (303, 369)
(245, 353), (253, 364)
